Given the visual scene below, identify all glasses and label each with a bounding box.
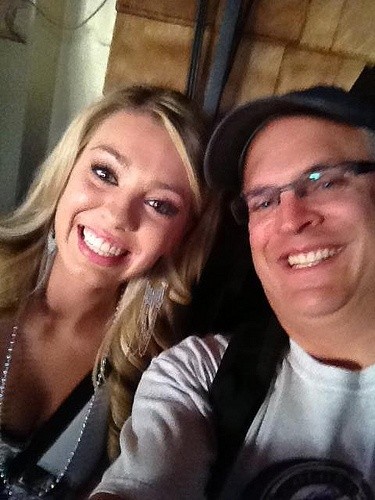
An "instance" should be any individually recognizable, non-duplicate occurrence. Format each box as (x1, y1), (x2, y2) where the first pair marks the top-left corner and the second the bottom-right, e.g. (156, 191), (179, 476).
(230, 161), (375, 225)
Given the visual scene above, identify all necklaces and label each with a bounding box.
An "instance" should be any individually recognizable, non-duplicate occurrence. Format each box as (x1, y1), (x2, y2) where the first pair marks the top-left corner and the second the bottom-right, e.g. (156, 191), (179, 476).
(0, 299), (106, 500)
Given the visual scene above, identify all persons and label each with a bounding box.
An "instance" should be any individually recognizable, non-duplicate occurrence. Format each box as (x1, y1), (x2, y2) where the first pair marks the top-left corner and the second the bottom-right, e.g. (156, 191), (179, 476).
(90, 85), (375, 500)
(0, 81), (217, 500)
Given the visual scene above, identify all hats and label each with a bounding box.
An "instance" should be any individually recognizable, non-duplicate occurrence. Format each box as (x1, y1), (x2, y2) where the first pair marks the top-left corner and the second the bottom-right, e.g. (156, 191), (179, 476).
(202, 86), (375, 227)
